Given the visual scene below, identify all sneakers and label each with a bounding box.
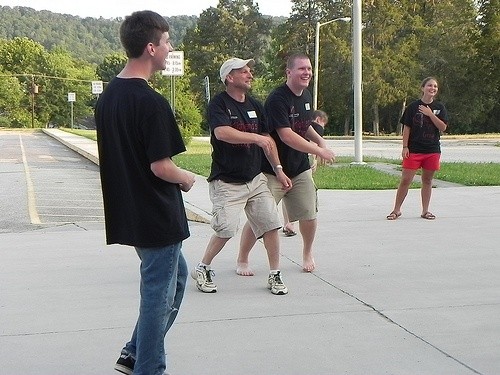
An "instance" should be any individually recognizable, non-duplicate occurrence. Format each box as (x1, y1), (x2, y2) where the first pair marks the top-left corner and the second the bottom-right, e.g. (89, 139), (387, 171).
(283, 223), (297, 236)
(267, 270), (288, 294)
(114, 353), (137, 375)
(190, 261), (217, 293)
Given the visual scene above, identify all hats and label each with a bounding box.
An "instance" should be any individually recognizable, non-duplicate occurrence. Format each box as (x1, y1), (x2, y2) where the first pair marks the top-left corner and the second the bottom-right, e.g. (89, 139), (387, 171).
(220, 58), (255, 85)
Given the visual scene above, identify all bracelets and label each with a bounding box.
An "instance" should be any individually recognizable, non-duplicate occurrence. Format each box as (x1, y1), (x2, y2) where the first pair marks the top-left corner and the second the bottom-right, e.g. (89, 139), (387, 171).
(272, 164), (283, 172)
(403, 146), (408, 149)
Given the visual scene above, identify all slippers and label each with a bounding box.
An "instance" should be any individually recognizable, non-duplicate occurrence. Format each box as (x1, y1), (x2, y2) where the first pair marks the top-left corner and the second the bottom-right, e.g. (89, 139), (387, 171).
(387, 211), (402, 220)
(421, 212), (436, 219)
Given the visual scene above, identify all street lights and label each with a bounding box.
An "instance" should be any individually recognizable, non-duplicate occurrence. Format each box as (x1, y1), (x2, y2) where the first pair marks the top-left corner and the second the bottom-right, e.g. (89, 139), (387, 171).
(314, 17), (351, 112)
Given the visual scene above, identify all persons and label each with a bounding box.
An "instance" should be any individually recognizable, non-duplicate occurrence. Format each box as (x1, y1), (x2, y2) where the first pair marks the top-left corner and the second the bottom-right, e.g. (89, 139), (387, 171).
(385, 76), (450, 221)
(234, 52), (337, 277)
(281, 108), (329, 237)
(93, 10), (197, 375)
(190, 56), (293, 295)
